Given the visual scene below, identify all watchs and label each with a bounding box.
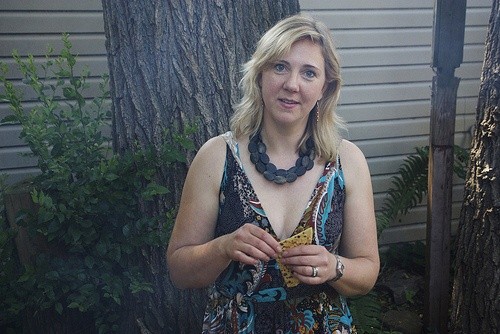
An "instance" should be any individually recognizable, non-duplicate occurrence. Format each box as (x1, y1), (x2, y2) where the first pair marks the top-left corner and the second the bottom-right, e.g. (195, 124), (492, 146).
(327, 254), (344, 282)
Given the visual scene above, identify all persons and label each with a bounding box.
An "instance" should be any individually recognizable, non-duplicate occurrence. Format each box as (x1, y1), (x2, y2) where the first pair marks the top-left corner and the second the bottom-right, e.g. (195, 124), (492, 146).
(166, 15), (380, 334)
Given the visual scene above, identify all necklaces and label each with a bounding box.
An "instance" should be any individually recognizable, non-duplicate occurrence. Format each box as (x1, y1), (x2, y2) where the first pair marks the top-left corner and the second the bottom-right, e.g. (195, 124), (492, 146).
(248, 127), (316, 184)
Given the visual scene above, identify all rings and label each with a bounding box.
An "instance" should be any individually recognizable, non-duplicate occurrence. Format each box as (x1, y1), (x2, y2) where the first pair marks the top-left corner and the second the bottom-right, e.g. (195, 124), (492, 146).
(311, 265), (318, 277)
